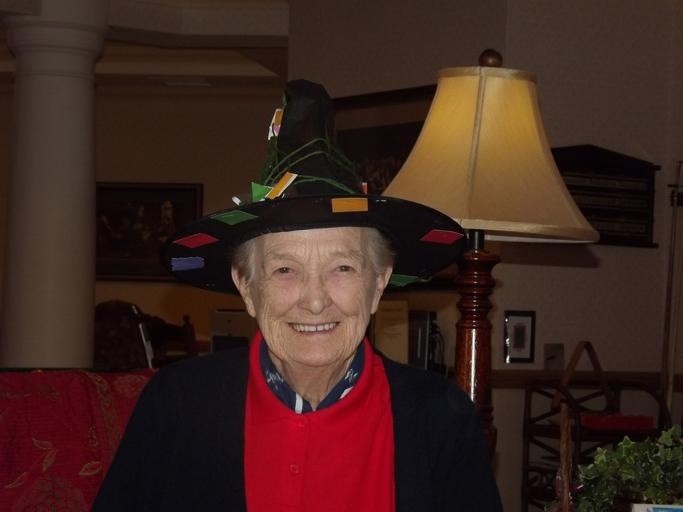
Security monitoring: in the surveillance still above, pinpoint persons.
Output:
[88,226,507,509]
[161,200,173,225]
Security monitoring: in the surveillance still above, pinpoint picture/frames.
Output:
[96,181,203,283]
[503,310,535,364]
[328,82,465,293]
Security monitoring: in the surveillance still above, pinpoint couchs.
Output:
[3,369,148,511]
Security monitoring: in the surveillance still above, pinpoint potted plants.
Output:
[575,423,682,511]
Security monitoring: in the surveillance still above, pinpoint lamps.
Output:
[372,48,603,466]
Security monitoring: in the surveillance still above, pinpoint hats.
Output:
[168,80,467,296]
